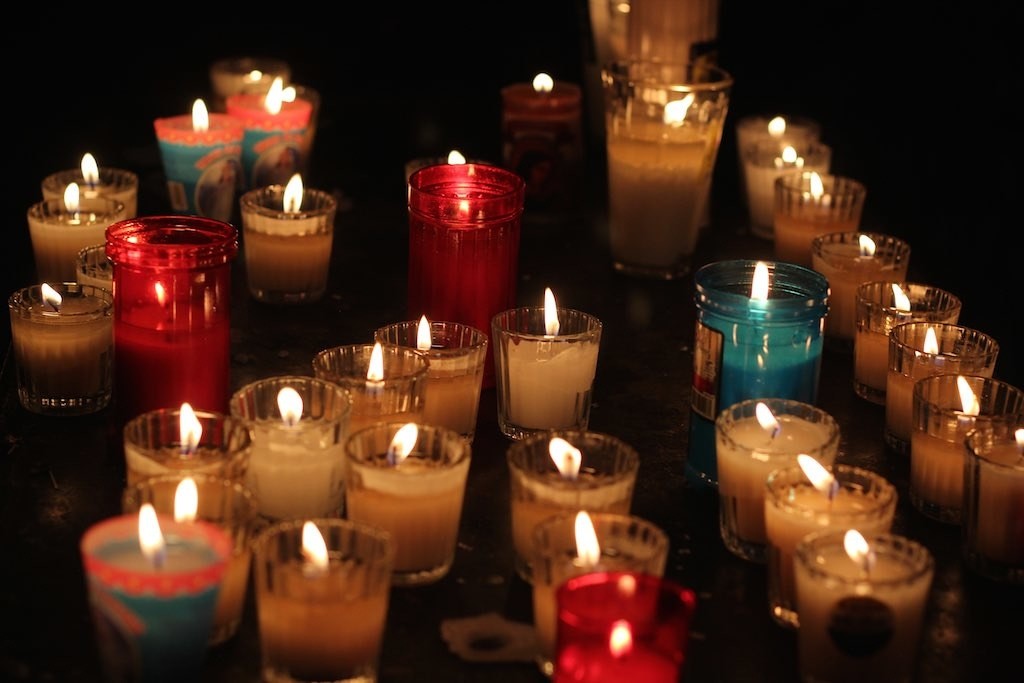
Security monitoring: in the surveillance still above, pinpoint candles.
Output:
[8,0,1024,683]
